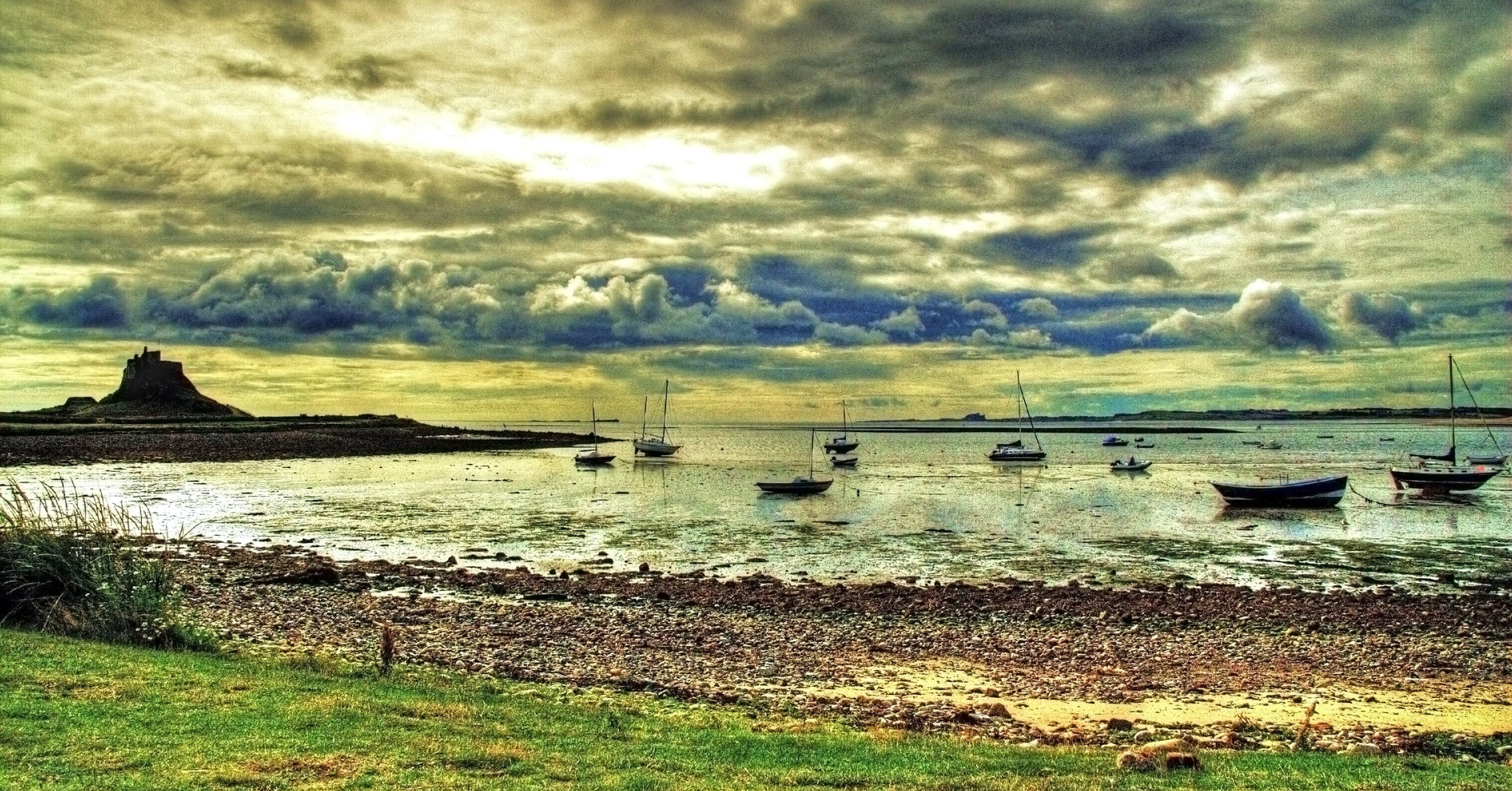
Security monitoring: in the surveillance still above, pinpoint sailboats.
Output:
[823,399,862,455]
[755,426,834,494]
[1389,352,1510,489]
[630,379,685,457]
[988,370,1047,462]
[575,401,617,464]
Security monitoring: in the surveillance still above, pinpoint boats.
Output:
[1109,454,1154,472]
[1242,441,1262,444]
[830,456,860,468]
[1465,455,1509,464]
[1379,438,1395,442]
[1134,443,1156,449]
[1187,437,1205,440]
[1208,474,1350,508]
[1101,436,1130,446]
[1261,440,1282,449]
[1317,434,1334,439]
[1134,437,1144,443]
